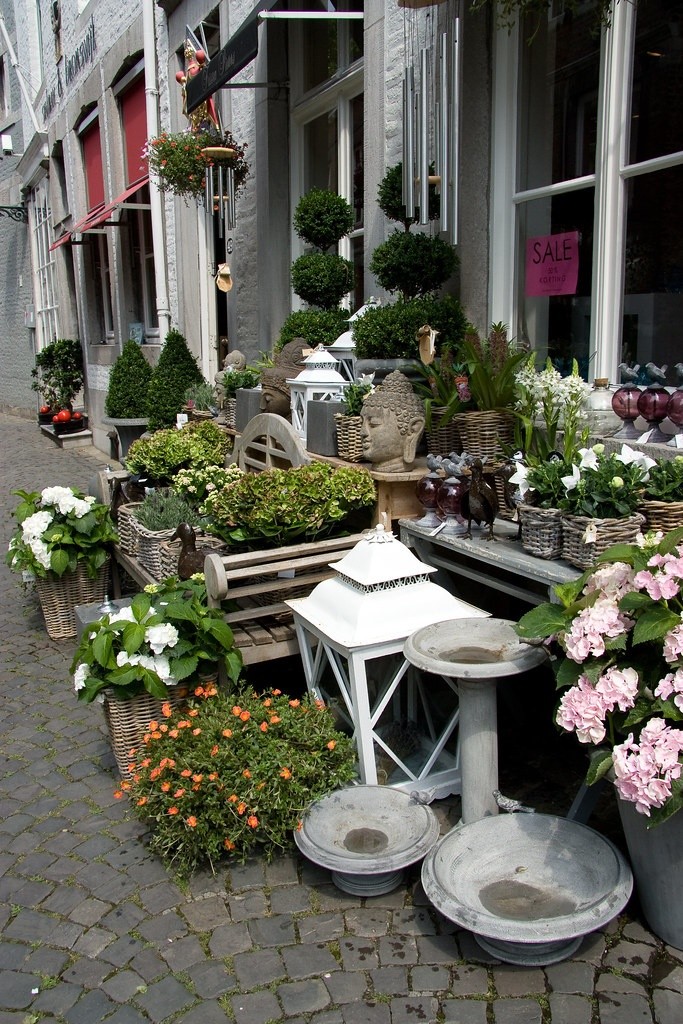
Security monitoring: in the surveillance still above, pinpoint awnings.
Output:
[185,0,364,116]
[47,173,149,250]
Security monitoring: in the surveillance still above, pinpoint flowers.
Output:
[135,124,253,210]
[3,320,683,892]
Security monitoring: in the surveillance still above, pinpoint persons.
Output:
[212,350,247,425]
[359,370,426,473]
[259,338,312,424]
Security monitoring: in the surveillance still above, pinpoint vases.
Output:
[332,411,368,464]
[558,509,645,572]
[99,672,220,786]
[420,405,463,460]
[450,402,522,463]
[634,496,683,548]
[159,535,224,583]
[222,396,236,430]
[606,715,683,952]
[34,553,111,643]
[512,498,565,562]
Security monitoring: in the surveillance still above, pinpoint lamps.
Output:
[284,343,356,450]
[636,362,675,444]
[323,295,385,383]
[665,363,683,447]
[281,519,497,805]
[611,362,644,439]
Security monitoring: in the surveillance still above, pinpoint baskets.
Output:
[560,511,646,571]
[517,501,564,560]
[634,489,683,545]
[192,409,214,422]
[159,537,226,582]
[452,406,516,464]
[118,502,142,556]
[492,462,519,521]
[425,407,463,458]
[181,406,196,421]
[35,552,112,641]
[130,518,203,582]
[224,398,236,429]
[333,412,365,462]
[101,671,218,782]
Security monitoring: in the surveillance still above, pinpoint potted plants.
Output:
[139,327,210,442]
[28,338,74,425]
[50,337,87,436]
[99,336,155,460]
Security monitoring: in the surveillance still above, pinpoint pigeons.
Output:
[107,476,153,525]
[456,458,501,541]
[172,522,250,590]
